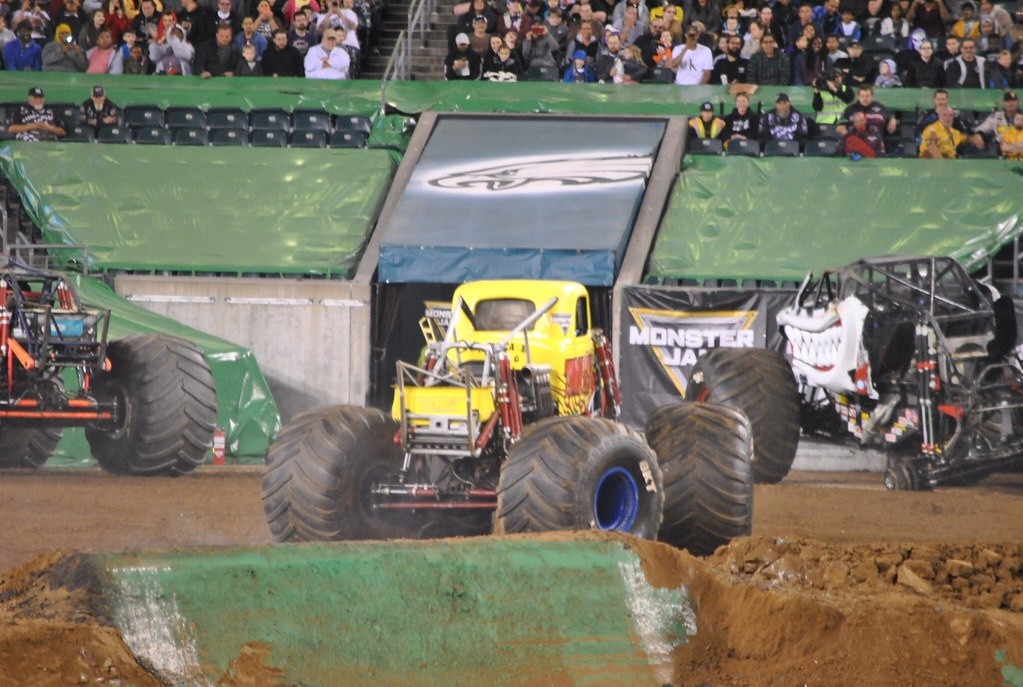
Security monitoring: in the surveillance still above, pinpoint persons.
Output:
[3,17,41,69]
[4,86,66,141]
[304,29,350,79]
[764,93,808,153]
[688,101,728,152]
[192,23,236,76]
[670,23,714,85]
[261,28,306,77]
[42,22,89,72]
[725,91,760,140]
[443,31,482,80]
[810,65,854,124]
[79,86,119,126]
[918,108,983,158]
[1,0,1023,158]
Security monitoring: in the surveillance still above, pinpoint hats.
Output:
[324,29,337,39]
[472,15,488,25]
[1004,91,1018,101]
[774,93,789,104]
[89,87,106,98]
[456,34,470,46]
[701,101,714,111]
[28,88,43,98]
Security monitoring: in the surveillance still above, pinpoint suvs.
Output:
[685,255,1022,490]
[0,260,219,478]
[260,278,755,558]
